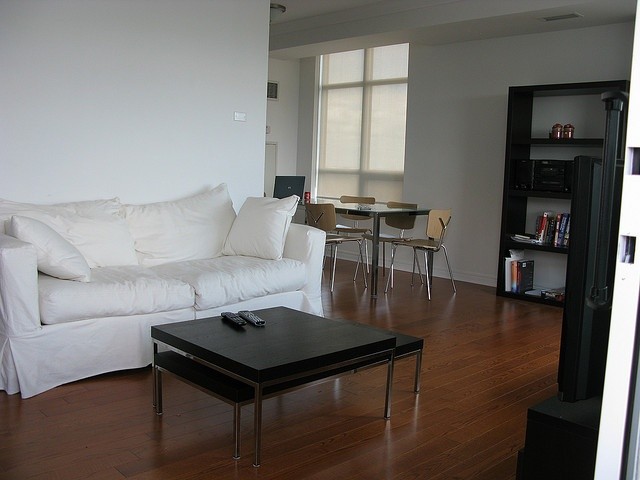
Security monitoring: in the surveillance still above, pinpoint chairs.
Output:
[305,203,370,292]
[355,201,425,294]
[385,209,458,302]
[337,195,374,282]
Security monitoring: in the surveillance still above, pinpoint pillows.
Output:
[0,199,139,268]
[3,215,92,283]
[123,183,236,270]
[223,194,300,261]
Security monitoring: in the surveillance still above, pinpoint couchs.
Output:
[0,181,327,397]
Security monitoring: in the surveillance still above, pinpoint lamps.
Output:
[270,3,286,26]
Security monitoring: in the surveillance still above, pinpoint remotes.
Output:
[221,312,247,326]
[238,311,266,327]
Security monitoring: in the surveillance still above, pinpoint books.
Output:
[506,210,570,248]
[505,257,534,294]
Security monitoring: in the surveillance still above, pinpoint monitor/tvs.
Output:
[556,155,604,401]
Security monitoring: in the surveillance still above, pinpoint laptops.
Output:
[273,175,305,201]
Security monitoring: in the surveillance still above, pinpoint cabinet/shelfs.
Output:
[514,393,601,479]
[493,78,630,311]
